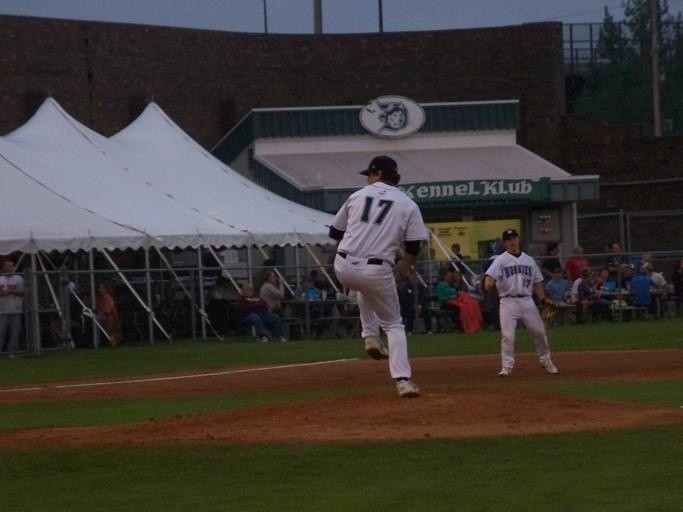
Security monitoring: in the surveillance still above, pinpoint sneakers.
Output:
[540,357,558,374]
[396,378,421,397]
[363,334,389,360]
[497,366,513,377]
[259,333,289,344]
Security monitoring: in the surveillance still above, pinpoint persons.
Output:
[208,270,325,342]
[326,153,428,398]
[477,229,558,377]
[50,272,141,348]
[543,242,682,323]
[1,258,25,358]
[397,243,496,336]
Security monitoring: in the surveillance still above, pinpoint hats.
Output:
[502,228,520,241]
[357,155,398,174]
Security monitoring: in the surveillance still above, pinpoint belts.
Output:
[338,250,383,266]
[505,292,527,298]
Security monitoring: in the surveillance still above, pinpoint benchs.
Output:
[228,289,664,340]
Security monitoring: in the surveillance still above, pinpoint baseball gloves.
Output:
[542,299,559,321]
